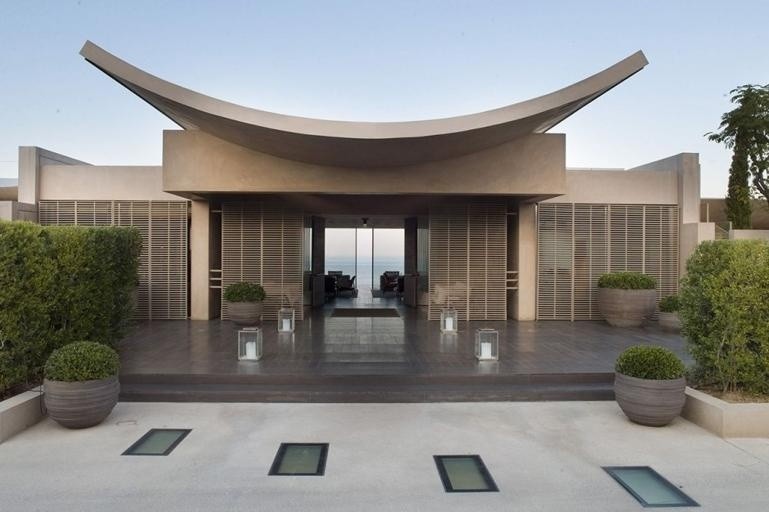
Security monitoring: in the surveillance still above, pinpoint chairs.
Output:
[325,271,356,297]
[380,271,406,301]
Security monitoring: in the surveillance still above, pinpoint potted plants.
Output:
[597,272,656,327]
[659,295,679,328]
[614,344,686,426]
[223,282,266,325]
[43,341,120,430]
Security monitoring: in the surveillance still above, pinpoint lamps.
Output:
[361,218,369,227]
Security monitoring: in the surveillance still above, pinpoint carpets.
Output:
[330,308,400,317]
[371,290,398,298]
[325,289,358,298]
